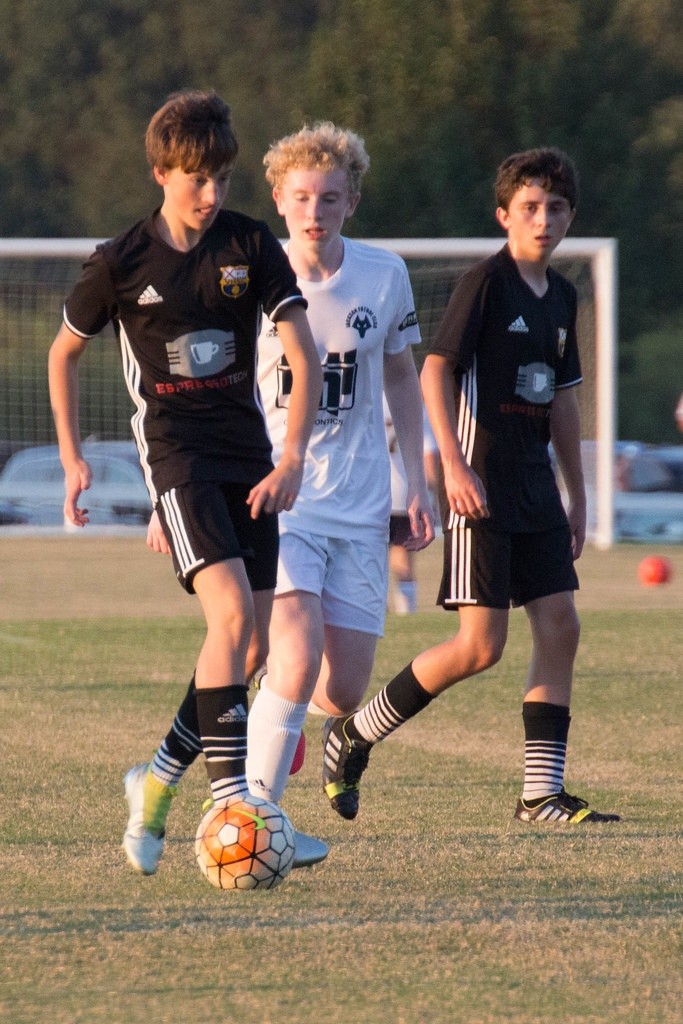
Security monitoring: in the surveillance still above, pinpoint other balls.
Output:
[636,553,675,587]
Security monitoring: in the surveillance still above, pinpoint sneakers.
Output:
[514,786,620,824]
[125,763,178,878]
[291,832,327,869]
[322,710,374,821]
[253,669,267,693]
[393,580,417,615]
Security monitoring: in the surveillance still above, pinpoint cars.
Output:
[0,436,153,527]
[646,445,683,481]
[556,442,683,544]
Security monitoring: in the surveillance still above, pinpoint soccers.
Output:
[191,791,298,895]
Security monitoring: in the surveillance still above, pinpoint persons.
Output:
[45,94,334,875]
[322,150,628,830]
[246,121,436,870]
